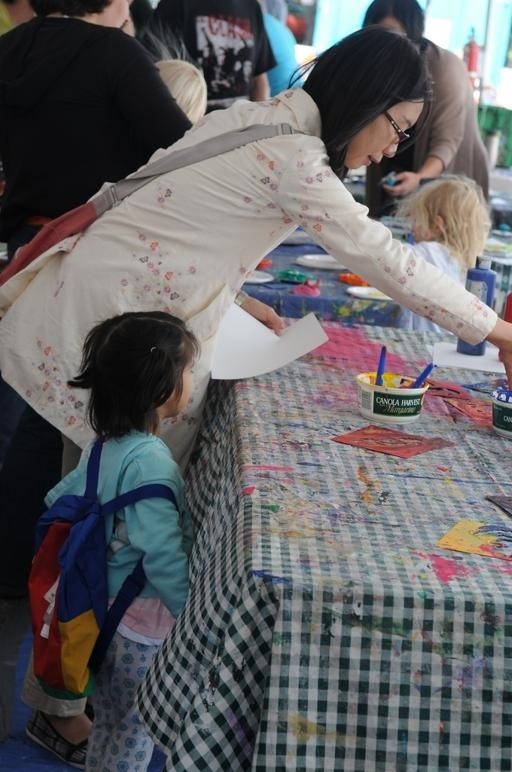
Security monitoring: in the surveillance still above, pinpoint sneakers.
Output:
[25,709,87,770]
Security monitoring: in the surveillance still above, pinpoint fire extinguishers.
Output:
[465,28,479,72]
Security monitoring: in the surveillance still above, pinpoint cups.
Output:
[355,372,429,423]
[491,397,511,440]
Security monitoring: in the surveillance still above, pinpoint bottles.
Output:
[456,257,496,356]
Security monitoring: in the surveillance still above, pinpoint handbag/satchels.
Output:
[1,201,97,287]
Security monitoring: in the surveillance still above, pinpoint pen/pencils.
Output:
[413,363,434,387]
[376,347,386,385]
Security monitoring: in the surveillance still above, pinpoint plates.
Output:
[244,270,274,284]
[345,283,391,302]
[295,251,347,271]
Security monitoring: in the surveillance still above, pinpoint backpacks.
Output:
[27,440,176,698]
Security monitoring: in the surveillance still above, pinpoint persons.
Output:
[44,310,201,772]
[394,173,491,332]
[0,0,302,265]
[357,0,489,221]
[0,29,512,767]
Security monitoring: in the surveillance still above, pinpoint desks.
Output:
[228,246,413,328]
[224,318,511,772]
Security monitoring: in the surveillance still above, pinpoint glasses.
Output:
[384,114,410,143]
